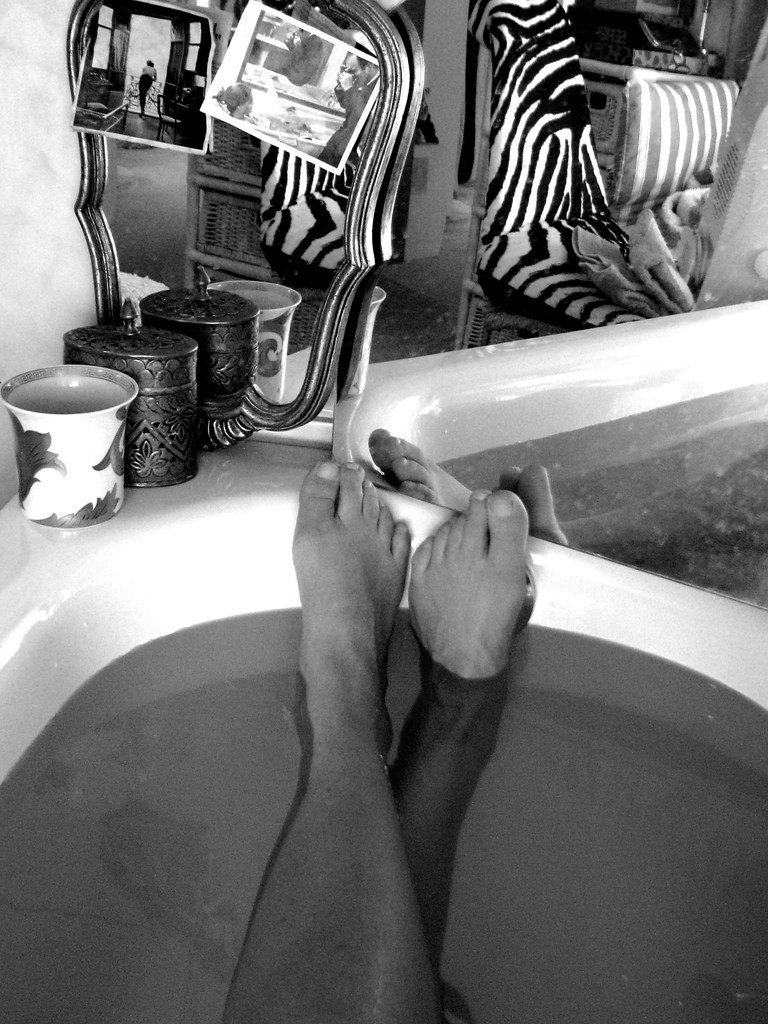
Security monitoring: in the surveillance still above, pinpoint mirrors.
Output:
[69,0,409,450]
[330,0,768,612]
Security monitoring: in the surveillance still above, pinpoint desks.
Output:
[77,94,130,135]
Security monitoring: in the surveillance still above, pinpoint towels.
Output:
[571,207,693,319]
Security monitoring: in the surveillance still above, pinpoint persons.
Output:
[368,428,768,592]
[283,26,324,87]
[222,457,529,1024]
[214,84,256,124]
[316,54,381,168]
[138,59,157,117]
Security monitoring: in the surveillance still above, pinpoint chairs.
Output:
[155,92,184,145]
[184,118,327,350]
[453,1,663,352]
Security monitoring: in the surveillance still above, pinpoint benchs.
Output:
[612,75,739,222]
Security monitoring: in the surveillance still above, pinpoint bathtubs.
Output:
[348,293,768,609]
[0,439,768,1024]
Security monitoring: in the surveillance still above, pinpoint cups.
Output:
[346,284,387,397]
[207,280,303,404]
[0,365,138,529]
